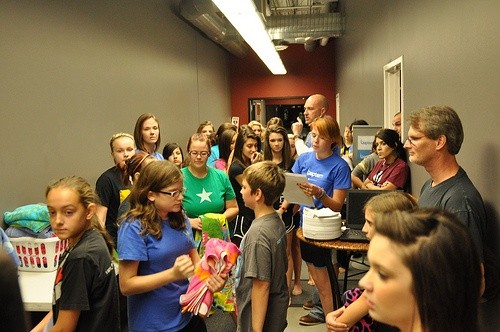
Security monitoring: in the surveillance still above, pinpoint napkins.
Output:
[308,207,339,218]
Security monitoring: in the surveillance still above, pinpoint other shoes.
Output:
[303,300,316,310]
[298,315,322,325]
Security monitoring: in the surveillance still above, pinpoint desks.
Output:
[296,227,370,310]
[18,262,121,332]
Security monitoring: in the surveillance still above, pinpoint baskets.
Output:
[3,235,69,271]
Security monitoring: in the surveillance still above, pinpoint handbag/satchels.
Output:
[344,287,374,332]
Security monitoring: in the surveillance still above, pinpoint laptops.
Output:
[341,189,405,240]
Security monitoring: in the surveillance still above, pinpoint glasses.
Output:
[407,135,432,146]
[158,187,186,197]
[189,150,210,157]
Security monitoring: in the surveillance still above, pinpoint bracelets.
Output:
[293,135,300,141]
[316,188,326,200]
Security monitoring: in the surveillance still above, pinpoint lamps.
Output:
[273,39,289,51]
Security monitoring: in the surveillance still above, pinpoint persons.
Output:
[117,152,158,216]
[162,143,184,169]
[133,113,164,162]
[392,112,401,138]
[115,161,229,332]
[362,128,408,190]
[229,129,262,247]
[341,120,368,158]
[292,94,329,156]
[403,105,485,259]
[261,124,303,295]
[199,116,284,169]
[351,129,386,189]
[180,132,239,239]
[325,191,419,332]
[288,133,316,286]
[235,161,290,332]
[358,207,483,332]
[93,133,136,248]
[291,115,352,325]
[29,176,120,332]
